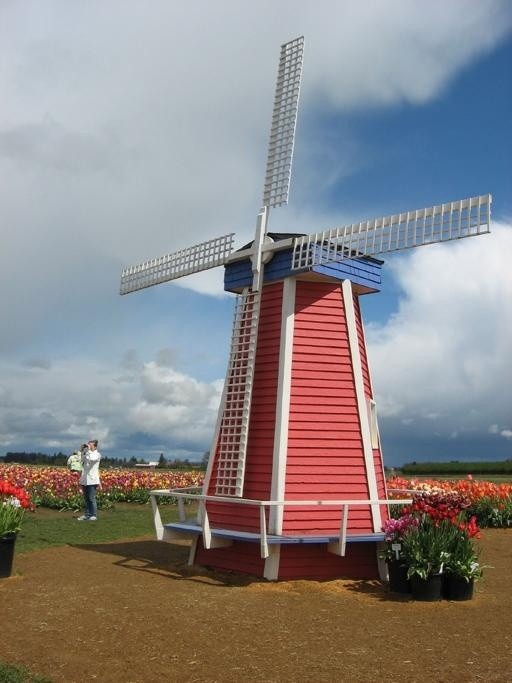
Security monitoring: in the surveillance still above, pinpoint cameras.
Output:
[82,444,88,448]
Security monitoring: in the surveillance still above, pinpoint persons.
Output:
[67,449,83,475]
[77,439,102,520]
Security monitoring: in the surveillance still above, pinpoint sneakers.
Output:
[77,514,98,521]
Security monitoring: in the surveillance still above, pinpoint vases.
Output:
[386,553,473,600]
[0,532,16,576]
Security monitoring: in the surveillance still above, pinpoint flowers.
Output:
[0,481,30,537]
[377,488,494,584]
